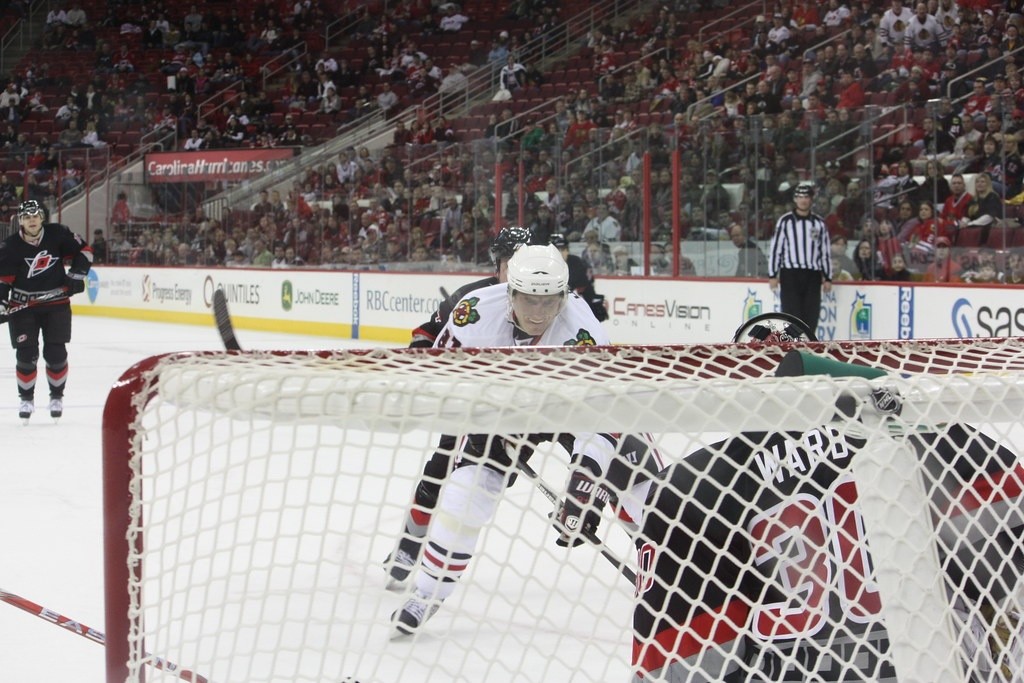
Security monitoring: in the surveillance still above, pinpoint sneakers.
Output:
[18,400,35,427]
[50,399,63,424]
[382,549,416,590]
[389,588,445,641]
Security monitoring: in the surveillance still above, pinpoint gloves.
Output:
[556,472,612,547]
[468,432,511,460]
[408,334,434,348]
[62,271,84,297]
[0,300,10,324]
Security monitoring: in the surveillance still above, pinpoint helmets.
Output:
[548,234,569,246]
[730,313,819,343]
[506,242,569,296]
[18,200,43,219]
[491,228,532,256]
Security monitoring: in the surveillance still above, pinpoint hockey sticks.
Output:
[206,288,243,351]
[1,592,208,683]
[504,442,638,589]
[0,285,71,317]
[438,287,458,311]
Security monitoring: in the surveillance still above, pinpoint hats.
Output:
[982,9,994,16]
[756,16,766,22]
[803,58,814,63]
[773,13,783,18]
[795,185,814,197]
[995,74,1003,79]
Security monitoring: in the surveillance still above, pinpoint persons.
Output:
[0,0,1024,279]
[0,201,92,417]
[770,187,831,339]
[384,225,664,633]
[632,313,1024,682]
